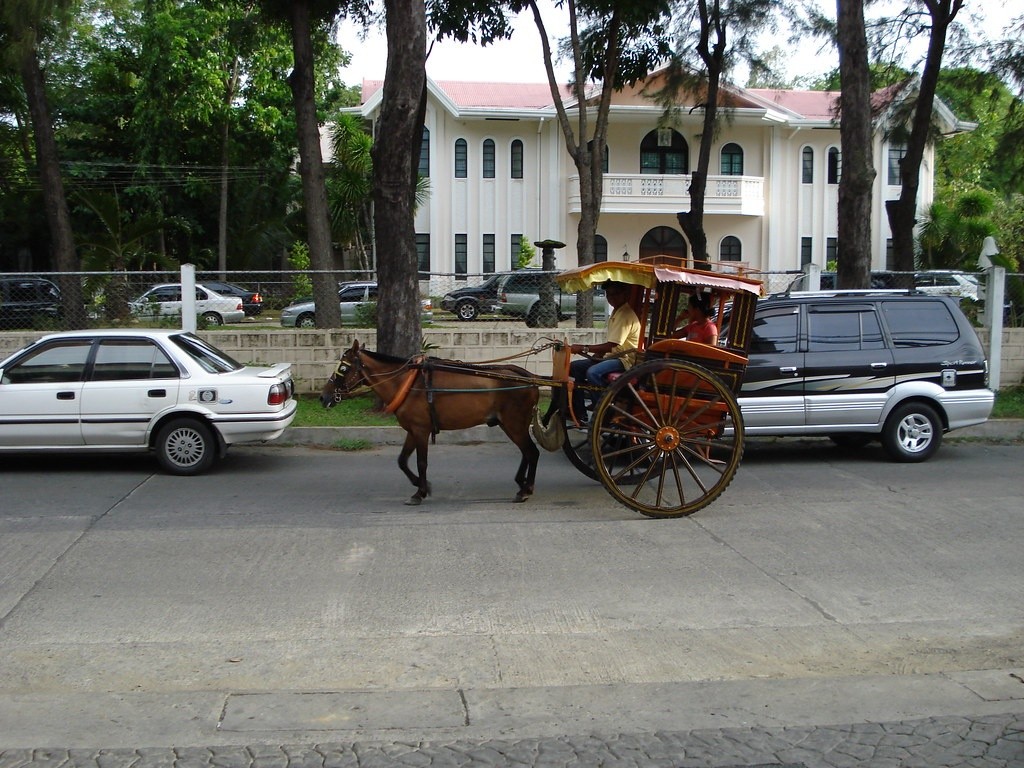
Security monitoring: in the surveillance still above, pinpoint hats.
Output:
[601,278,631,289]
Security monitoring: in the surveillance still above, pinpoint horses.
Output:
[319,339,541,506]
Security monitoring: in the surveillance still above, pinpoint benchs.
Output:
[606,372,639,387]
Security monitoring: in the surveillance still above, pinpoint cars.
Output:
[278,280,433,330]
[124,284,246,329]
[199,280,263,317]
[0,328,297,476]
[914,268,986,302]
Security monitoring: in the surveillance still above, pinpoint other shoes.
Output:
[586,403,594,412]
[575,412,589,421]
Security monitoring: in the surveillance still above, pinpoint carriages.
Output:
[318,255,766,521]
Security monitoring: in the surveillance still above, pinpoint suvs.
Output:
[488,268,610,328]
[0,274,69,329]
[575,288,995,469]
[438,271,514,321]
[786,267,910,294]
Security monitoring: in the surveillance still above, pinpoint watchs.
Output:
[582,345,589,354]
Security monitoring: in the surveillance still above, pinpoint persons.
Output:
[669,292,718,348]
[569,278,644,421]
